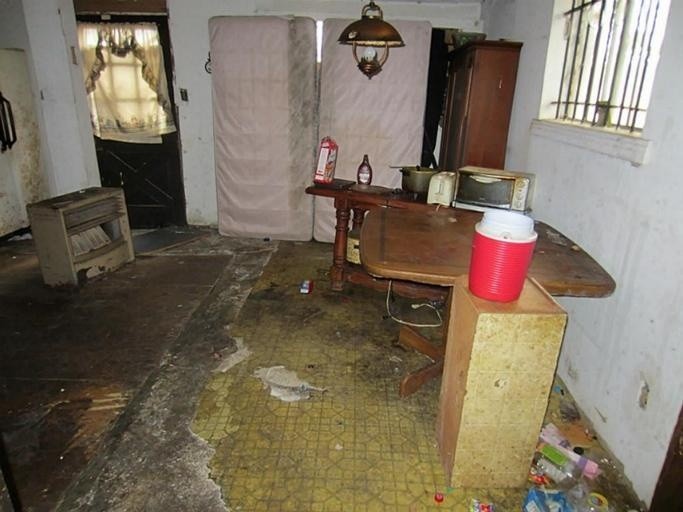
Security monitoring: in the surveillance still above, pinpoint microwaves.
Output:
[450,164,536,218]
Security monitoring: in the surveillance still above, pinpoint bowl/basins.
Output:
[450,31,486,49]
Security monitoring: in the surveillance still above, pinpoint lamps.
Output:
[337,0,405,81]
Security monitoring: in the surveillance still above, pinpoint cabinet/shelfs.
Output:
[27,187,135,290]
[438,40,522,171]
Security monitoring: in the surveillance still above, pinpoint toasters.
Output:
[425,170,456,208]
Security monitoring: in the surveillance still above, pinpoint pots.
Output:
[400,164,439,195]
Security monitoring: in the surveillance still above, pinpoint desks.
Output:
[306,178,449,306]
[361,208,616,399]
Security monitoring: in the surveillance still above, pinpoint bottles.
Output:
[355,154,372,185]
[531,437,582,490]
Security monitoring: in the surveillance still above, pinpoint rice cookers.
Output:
[344,230,362,266]
